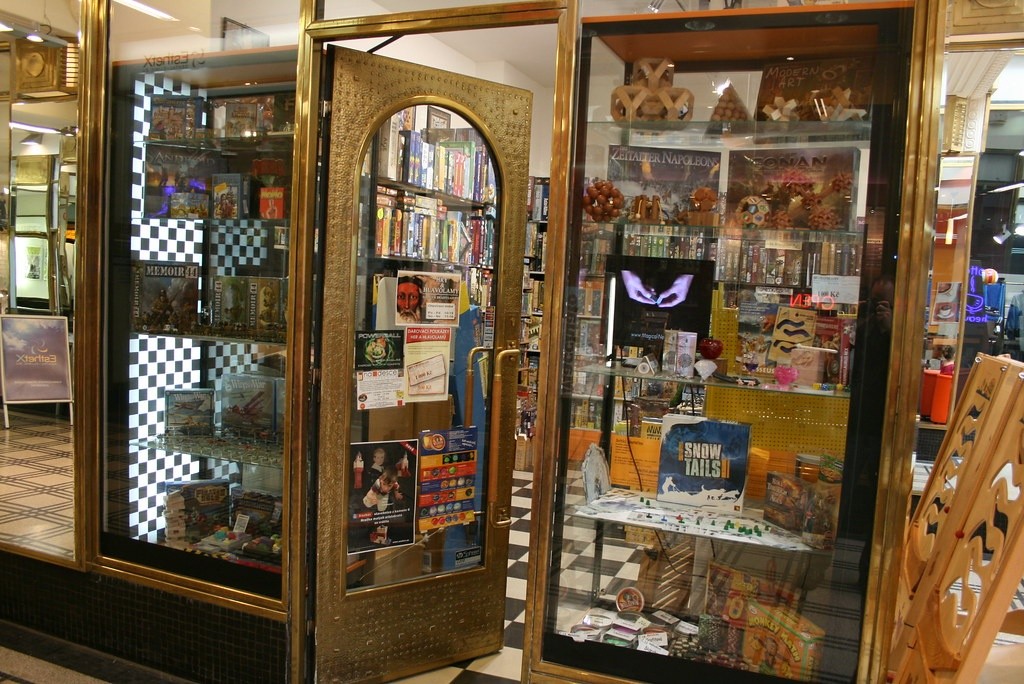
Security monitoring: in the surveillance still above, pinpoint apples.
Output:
[700,335,723,359]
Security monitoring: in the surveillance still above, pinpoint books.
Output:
[516,174,862,550]
[354,106,497,411]
[349,426,483,569]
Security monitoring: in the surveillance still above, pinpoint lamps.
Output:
[19,132,45,146]
[13,35,79,100]
[993,223,1011,245]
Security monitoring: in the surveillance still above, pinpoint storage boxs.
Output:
[149,87,297,139]
[706,564,826,682]
[169,173,286,221]
[764,471,814,529]
[131,259,282,569]
[363,520,480,583]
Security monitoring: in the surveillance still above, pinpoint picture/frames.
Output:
[222,16,272,51]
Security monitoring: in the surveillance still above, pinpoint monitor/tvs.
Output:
[598,253,716,367]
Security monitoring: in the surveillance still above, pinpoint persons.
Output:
[1004,295,1024,357]
[930,345,956,377]
[618,259,699,308]
[368,449,386,483]
[363,469,403,513]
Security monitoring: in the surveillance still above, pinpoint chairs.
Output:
[580,443,662,605]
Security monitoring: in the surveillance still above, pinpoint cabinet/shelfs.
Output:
[10,99,78,343]
[914,150,1024,429]
[360,113,869,502]
[99,42,298,605]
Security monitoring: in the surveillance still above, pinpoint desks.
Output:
[575,488,813,627]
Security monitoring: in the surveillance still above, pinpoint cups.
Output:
[795,453,822,483]
[931,373,952,423]
[661,327,698,378]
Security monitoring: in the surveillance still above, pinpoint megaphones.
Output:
[659,328,697,376]
